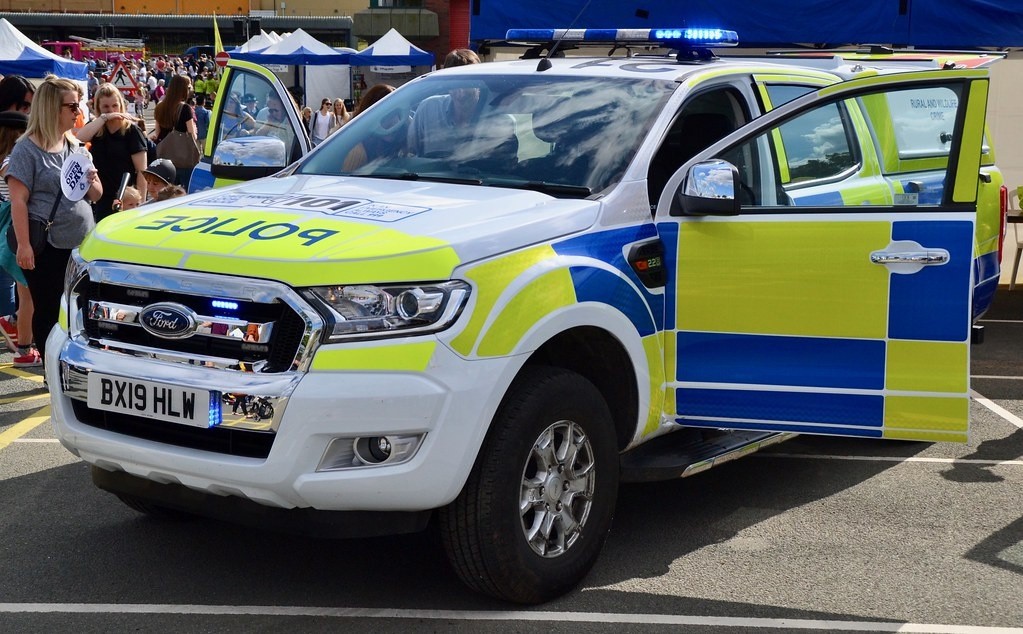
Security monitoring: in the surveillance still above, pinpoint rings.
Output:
[19,262,21,263]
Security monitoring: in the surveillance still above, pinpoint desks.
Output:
[1007,209,1023,223]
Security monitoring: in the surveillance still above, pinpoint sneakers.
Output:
[0,317,19,352]
[14,348,43,367]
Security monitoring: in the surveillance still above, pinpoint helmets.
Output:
[141,158,176,186]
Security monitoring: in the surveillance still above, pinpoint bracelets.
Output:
[101,113,108,121]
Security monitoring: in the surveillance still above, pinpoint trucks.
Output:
[40,35,146,63]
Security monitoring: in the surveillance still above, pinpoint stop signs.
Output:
[215,51,230,67]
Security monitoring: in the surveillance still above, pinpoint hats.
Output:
[243,93,257,103]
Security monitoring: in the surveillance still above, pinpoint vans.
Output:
[178,45,236,64]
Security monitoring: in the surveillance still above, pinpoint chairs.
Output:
[645,111,703,205]
[507,105,578,182]
[1008,191,1023,290]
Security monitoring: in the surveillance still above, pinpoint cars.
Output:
[44,45,1009,604]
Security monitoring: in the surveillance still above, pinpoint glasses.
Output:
[61,103,79,113]
[325,103,332,106]
[268,109,279,114]
[19,101,31,110]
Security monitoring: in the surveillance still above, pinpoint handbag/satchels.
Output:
[156,103,200,169]
[7,212,48,257]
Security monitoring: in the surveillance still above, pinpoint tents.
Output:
[225,26,436,110]
[0,18,90,124]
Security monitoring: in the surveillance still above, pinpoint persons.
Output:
[218,83,413,173]
[409,47,518,158]
[0,50,225,368]
